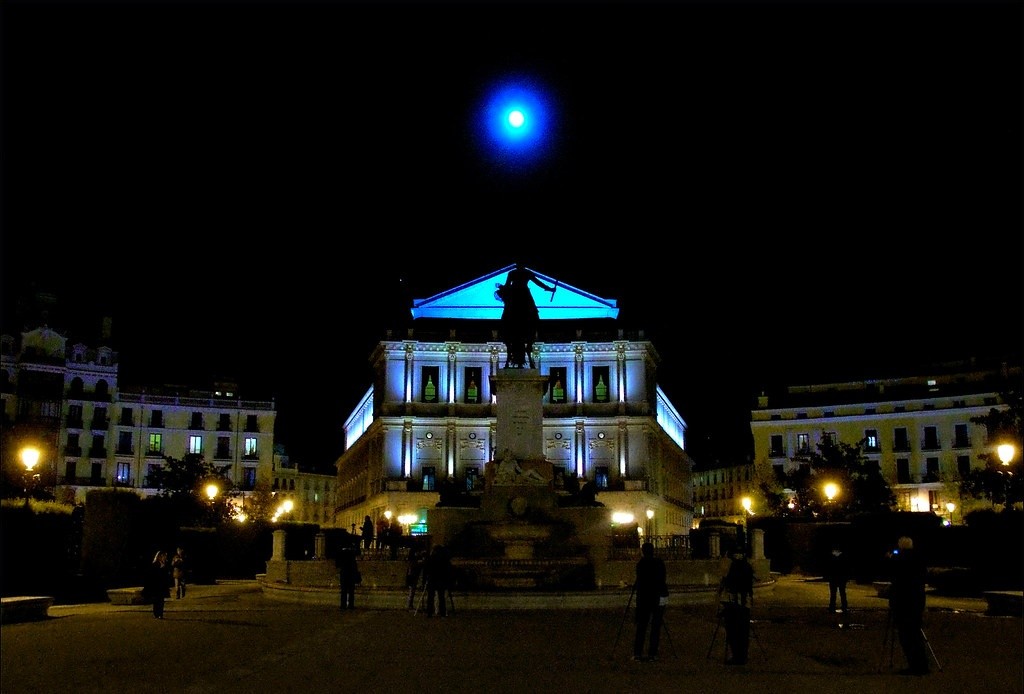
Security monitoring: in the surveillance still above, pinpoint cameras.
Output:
[892,550,899,555]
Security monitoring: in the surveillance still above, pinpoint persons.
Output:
[499,449,552,483]
[721,547,754,666]
[823,548,850,619]
[630,542,667,663]
[151,548,187,620]
[335,516,451,618]
[505,263,556,351]
[885,536,931,676]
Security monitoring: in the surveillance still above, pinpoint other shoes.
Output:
[630,656,642,665]
[647,656,655,664]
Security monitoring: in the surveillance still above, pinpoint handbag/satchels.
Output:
[406,575,417,585]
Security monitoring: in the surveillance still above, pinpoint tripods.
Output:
[707,619,767,666]
[609,587,678,660]
[413,580,455,616]
[878,607,942,675]
